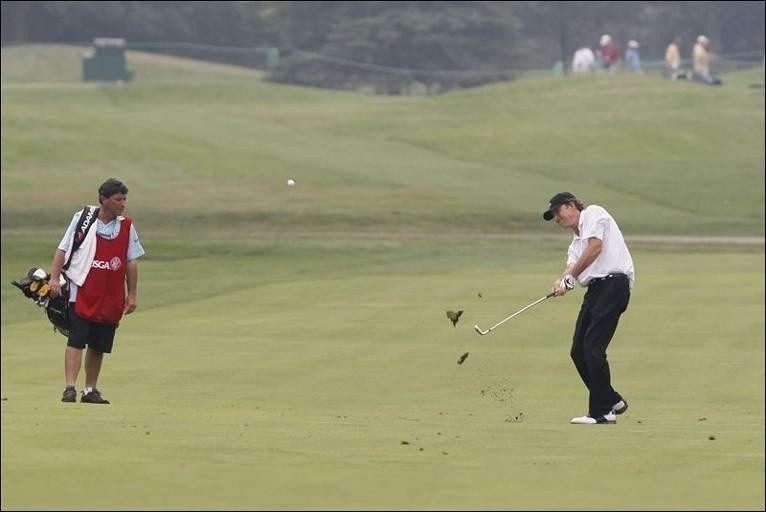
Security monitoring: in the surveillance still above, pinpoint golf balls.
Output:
[286,179,295,187]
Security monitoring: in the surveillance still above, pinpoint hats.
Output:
[543,192,578,221]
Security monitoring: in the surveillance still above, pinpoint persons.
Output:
[45,178,144,404]
[571,32,716,89]
[543,192,636,424]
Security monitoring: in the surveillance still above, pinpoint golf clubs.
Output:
[475,291,556,335]
[12,264,67,309]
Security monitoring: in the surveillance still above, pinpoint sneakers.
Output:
[571,400,628,425]
[61,386,76,402]
[81,387,109,404]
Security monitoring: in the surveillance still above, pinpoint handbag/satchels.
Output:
[46,292,70,332]
